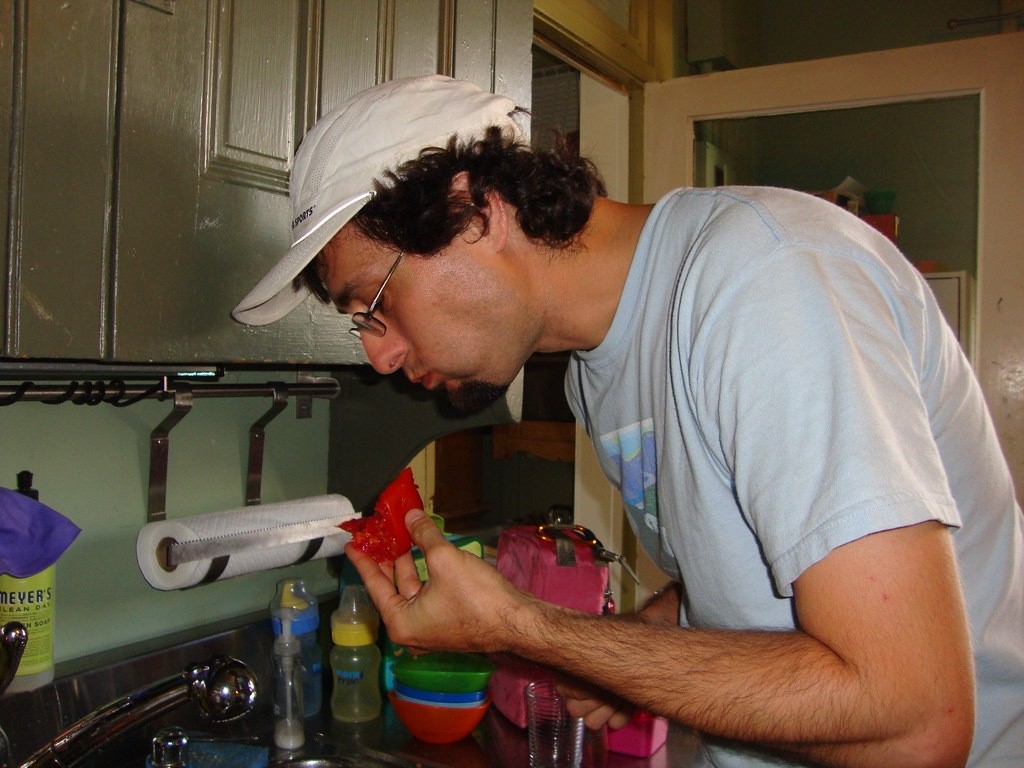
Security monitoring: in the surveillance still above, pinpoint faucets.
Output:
[16,657,259,767]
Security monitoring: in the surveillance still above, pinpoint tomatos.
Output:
[337,467,427,563]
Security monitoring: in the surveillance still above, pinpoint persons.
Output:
[234,75,1024,768]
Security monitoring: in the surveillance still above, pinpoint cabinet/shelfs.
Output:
[0,0,533,386]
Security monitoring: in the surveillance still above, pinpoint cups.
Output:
[525,680,607,768]
[864,188,898,214]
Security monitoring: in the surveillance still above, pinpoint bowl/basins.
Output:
[388,659,494,744]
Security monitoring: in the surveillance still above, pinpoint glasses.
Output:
[348,249,408,341]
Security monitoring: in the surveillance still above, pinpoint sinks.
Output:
[266,736,434,768]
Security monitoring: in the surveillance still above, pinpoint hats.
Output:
[230,75,524,327]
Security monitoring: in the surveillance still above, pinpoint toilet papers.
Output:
[137,493,364,591]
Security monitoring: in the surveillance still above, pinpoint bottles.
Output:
[268,578,322,719]
[0,470,57,695]
[269,607,306,751]
[328,585,383,723]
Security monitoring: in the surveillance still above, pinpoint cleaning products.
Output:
[0,470,58,694]
